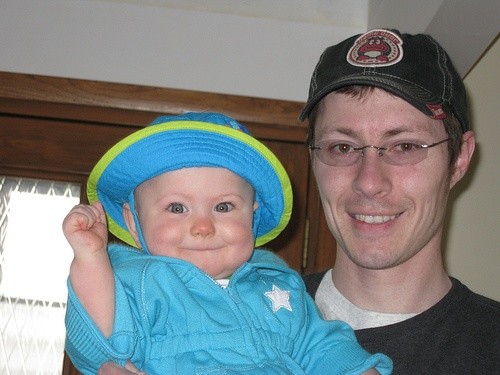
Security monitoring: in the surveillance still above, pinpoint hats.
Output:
[298,29,469,133]
[86,112,294,251]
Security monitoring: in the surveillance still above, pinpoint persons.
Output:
[62,111,396,375]
[94,28,500,374]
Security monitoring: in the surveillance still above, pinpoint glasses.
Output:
[308,138,450,167]
